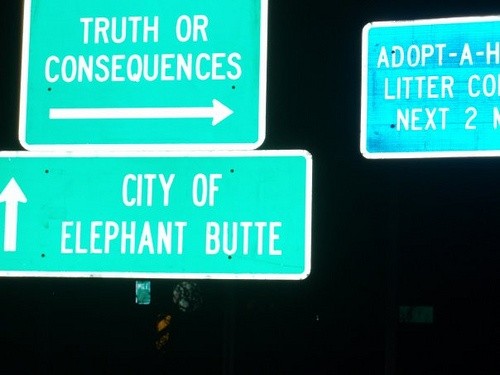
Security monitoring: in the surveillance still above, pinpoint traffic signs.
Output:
[18,1,268,149]
[359,16,500,161]
[0,149,312,280]
